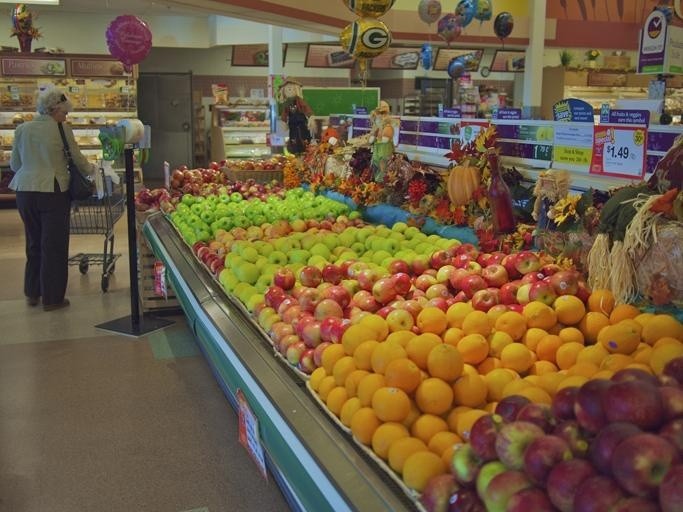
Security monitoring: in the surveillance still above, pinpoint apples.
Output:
[423,356,683,512]
[134,155,592,374]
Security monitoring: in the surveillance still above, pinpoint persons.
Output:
[8,86,93,311]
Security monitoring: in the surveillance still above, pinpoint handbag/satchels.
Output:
[69,164,96,201]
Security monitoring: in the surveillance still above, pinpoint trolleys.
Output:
[66,168,124,293]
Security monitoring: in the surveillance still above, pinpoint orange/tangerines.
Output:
[310,287,683,492]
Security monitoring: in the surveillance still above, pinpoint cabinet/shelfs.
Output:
[209,99,274,172]
[0,75,150,209]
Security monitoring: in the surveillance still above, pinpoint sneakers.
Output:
[26,295,41,305]
[42,299,70,311]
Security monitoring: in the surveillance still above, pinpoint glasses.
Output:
[57,94,67,105]
